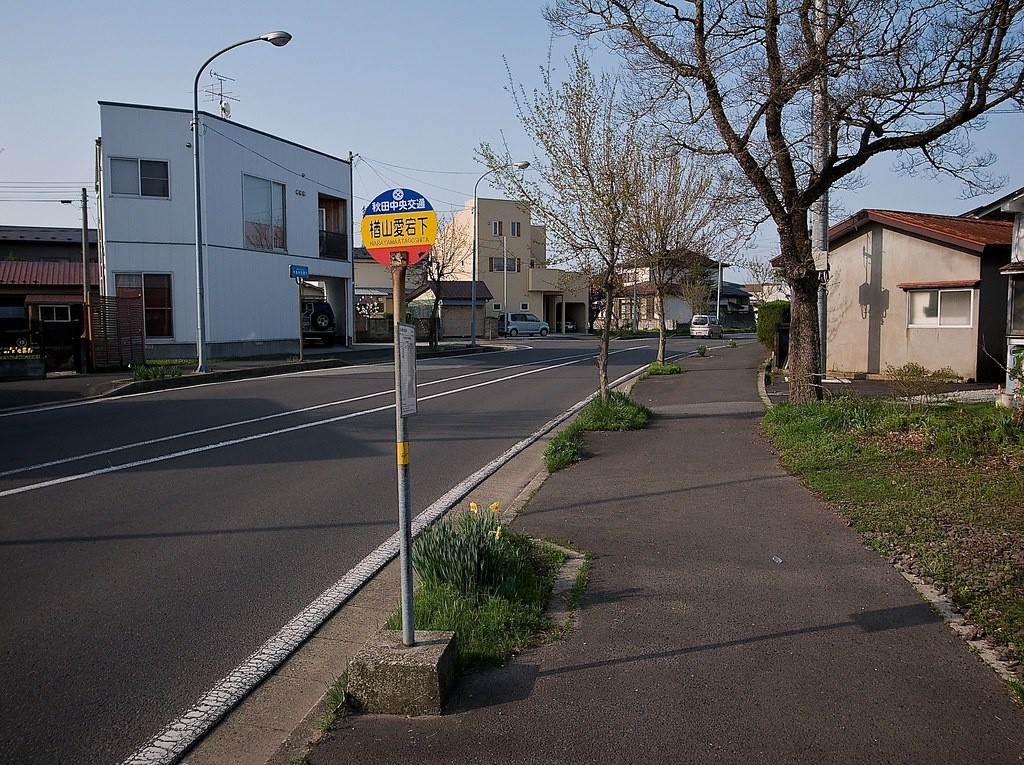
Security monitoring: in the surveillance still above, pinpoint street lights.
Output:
[193,30,292,374]
[717,237,751,321]
[472,161,531,347]
[61,200,94,373]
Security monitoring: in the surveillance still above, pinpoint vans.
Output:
[497,312,549,336]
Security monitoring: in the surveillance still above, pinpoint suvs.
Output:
[301,296,337,346]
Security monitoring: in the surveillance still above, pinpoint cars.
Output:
[689,315,724,339]
[557,319,578,333]
